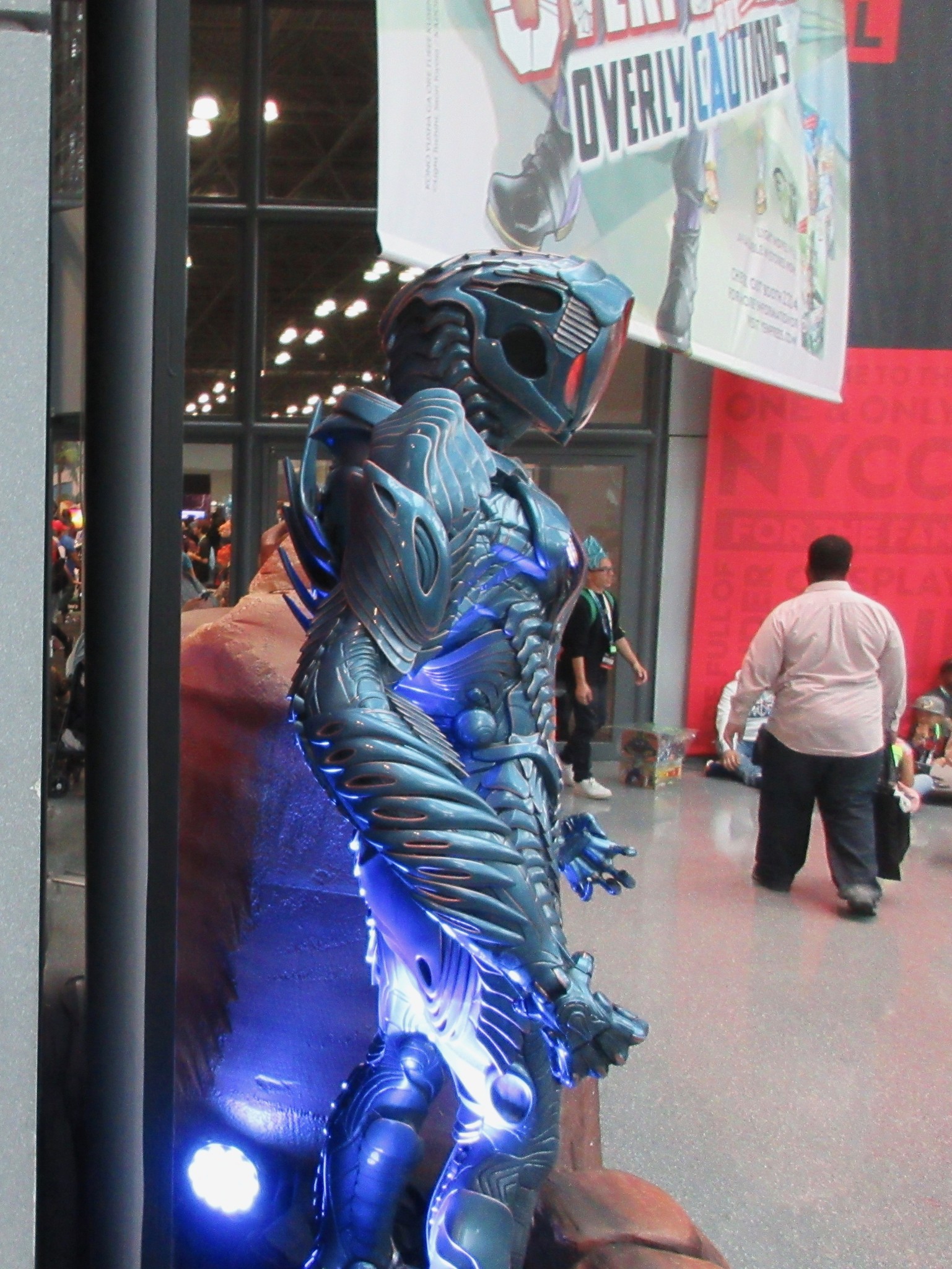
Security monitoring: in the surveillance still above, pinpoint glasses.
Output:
[590,564,613,573]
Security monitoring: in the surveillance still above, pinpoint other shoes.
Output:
[705,759,724,776]
[753,864,794,891]
[851,891,879,917]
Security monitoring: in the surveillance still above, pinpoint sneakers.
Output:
[558,757,612,799]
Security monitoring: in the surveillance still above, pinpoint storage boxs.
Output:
[617,727,683,791]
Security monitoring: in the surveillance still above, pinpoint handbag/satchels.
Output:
[875,787,912,881]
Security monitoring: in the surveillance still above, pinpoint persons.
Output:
[873,658,952,816]
[556,552,648,799]
[723,535,906,917]
[44,497,86,797]
[278,249,649,1269]
[704,668,776,788]
[276,500,292,523]
[181,505,233,609]
